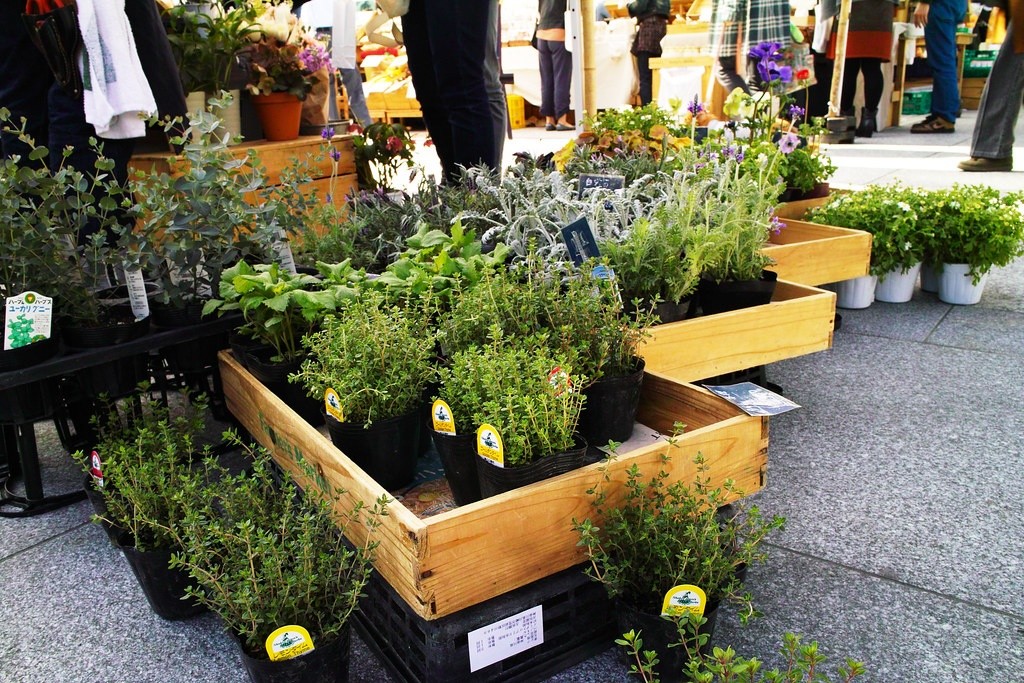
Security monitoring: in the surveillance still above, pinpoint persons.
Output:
[825,0,896,138]
[382,0,506,190]
[957,0,1024,174]
[625,0,670,109]
[706,0,792,96]
[534,0,576,133]
[300,0,373,131]
[910,0,968,134]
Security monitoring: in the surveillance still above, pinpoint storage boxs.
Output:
[963,49,1000,78]
[216,346,768,620]
[506,95,525,130]
[901,92,932,115]
[688,365,767,391]
[775,188,850,220]
[255,442,629,683]
[961,78,986,109]
[632,279,836,384]
[759,216,873,287]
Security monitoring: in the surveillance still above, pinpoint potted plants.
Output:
[0,0,865,683]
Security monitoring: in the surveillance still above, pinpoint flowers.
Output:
[245,16,1024,287]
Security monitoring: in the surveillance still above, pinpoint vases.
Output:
[251,91,302,142]
[825,261,988,305]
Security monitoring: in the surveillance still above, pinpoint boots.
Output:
[855,106,878,138]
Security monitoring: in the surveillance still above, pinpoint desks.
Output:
[853,22,925,128]
[914,32,978,100]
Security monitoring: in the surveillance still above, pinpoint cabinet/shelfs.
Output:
[127,134,358,256]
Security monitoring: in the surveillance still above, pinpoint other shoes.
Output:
[546,123,576,131]
[910,113,955,133]
[957,157,1013,172]
